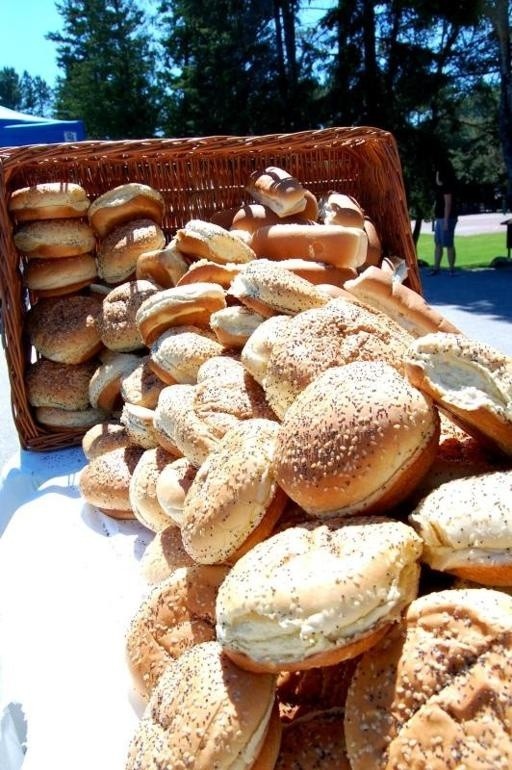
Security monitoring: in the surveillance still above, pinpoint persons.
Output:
[425,156,458,277]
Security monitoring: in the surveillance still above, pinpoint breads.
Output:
[7,165,512,770]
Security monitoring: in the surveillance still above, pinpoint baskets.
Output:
[0,125,423,452]
